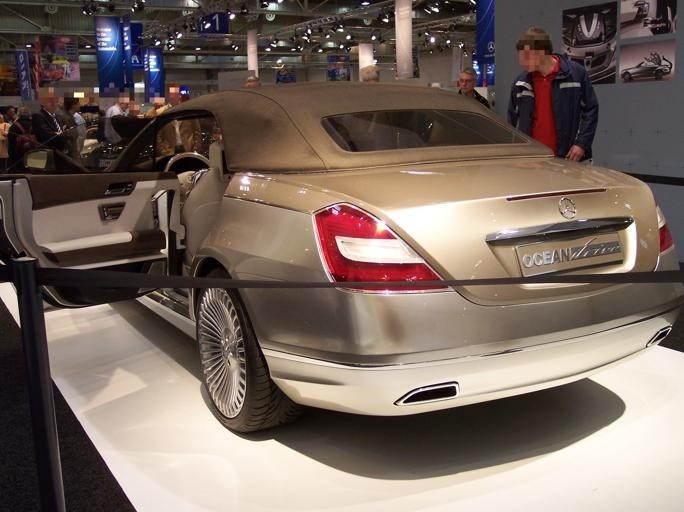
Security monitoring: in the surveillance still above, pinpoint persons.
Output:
[327,55,350,81]
[457,67,490,109]
[359,65,380,84]
[646,53,655,64]
[0,75,264,175]
[62,60,71,78]
[505,26,599,167]
[653,53,662,65]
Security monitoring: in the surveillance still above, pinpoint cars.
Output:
[560,1,673,81]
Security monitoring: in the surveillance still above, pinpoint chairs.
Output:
[180,140,234,267]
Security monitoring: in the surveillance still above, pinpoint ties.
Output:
[53,117,61,131]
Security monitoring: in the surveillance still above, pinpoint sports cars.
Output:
[1,79,681,442]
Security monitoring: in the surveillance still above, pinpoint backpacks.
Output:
[15,122,37,153]
